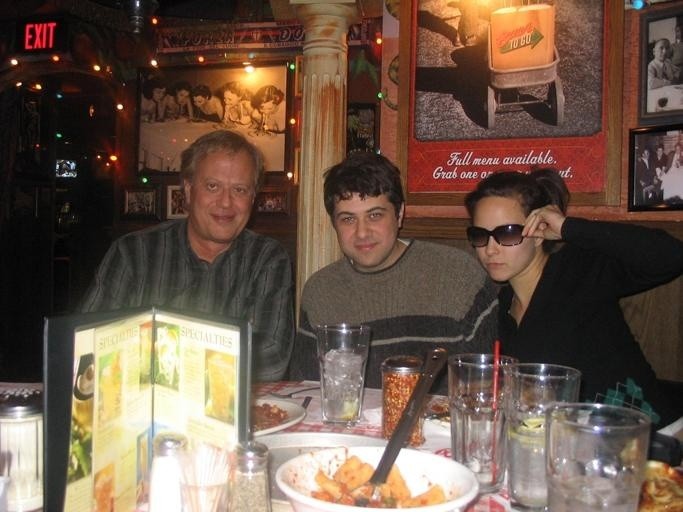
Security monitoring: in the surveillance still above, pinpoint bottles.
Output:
[233,439,271,511]
[149,433,186,511]
[381,354,425,448]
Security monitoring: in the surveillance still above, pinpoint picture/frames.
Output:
[639,4,683,127]
[347,100,380,159]
[122,187,163,221]
[629,124,683,211]
[396,1,625,208]
[134,56,294,178]
[253,185,297,219]
[163,182,189,219]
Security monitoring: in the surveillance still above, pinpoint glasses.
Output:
[465,223,526,248]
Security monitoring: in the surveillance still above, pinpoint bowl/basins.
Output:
[275,445,480,512]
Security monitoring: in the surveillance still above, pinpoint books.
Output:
[42,305,252,512]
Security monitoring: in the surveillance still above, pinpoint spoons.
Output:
[352,345,449,502]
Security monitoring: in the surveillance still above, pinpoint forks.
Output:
[267,386,322,399]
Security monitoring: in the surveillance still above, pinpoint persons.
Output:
[257,193,288,212]
[465,167,683,430]
[191,84,224,122]
[668,24,683,66]
[636,148,656,205]
[251,85,285,133]
[126,192,153,214]
[167,81,194,119]
[648,38,683,90]
[652,145,669,177]
[76,130,295,383]
[172,192,187,215]
[140,77,167,122]
[289,149,497,396]
[667,143,683,173]
[222,80,254,127]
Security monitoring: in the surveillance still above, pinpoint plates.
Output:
[252,430,390,506]
[250,397,307,437]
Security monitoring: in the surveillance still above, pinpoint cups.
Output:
[317,322,372,424]
[444,352,520,495]
[543,403,652,512]
[502,363,582,511]
